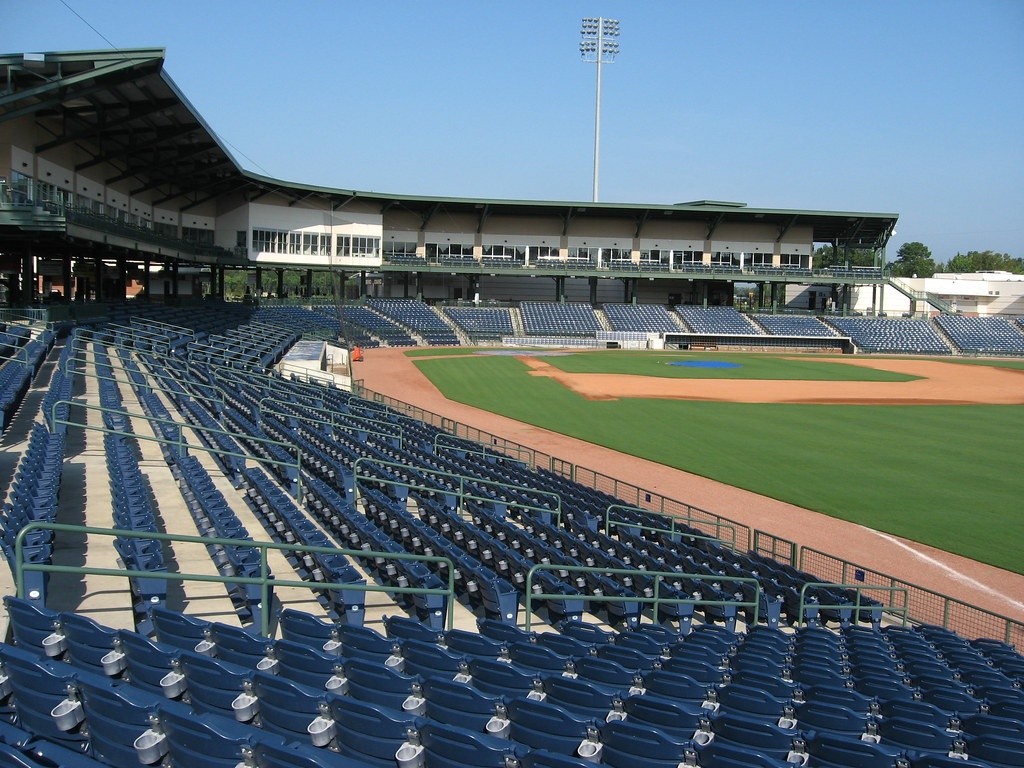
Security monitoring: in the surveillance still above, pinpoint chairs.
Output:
[0,184,1024,767]
